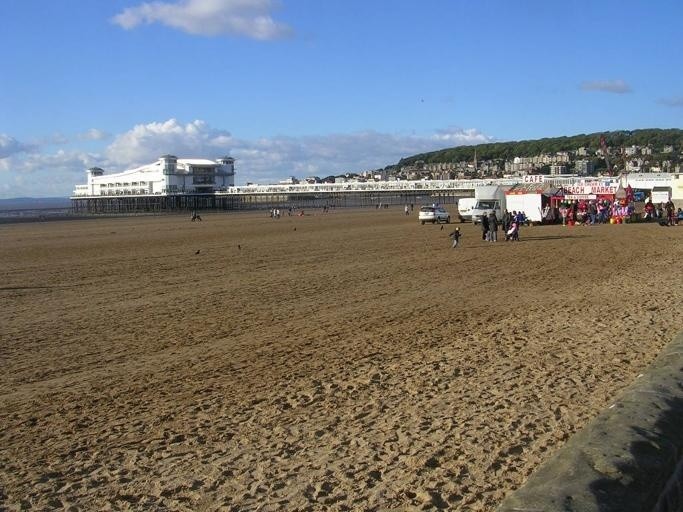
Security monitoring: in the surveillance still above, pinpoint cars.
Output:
[418,205,451,225]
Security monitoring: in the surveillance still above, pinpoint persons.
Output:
[480,208,527,240]
[374,201,389,209]
[410,200,415,211]
[271,206,306,218]
[542,199,683,226]
[449,227,464,248]
[404,203,409,217]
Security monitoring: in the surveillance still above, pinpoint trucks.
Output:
[471,185,506,225]
